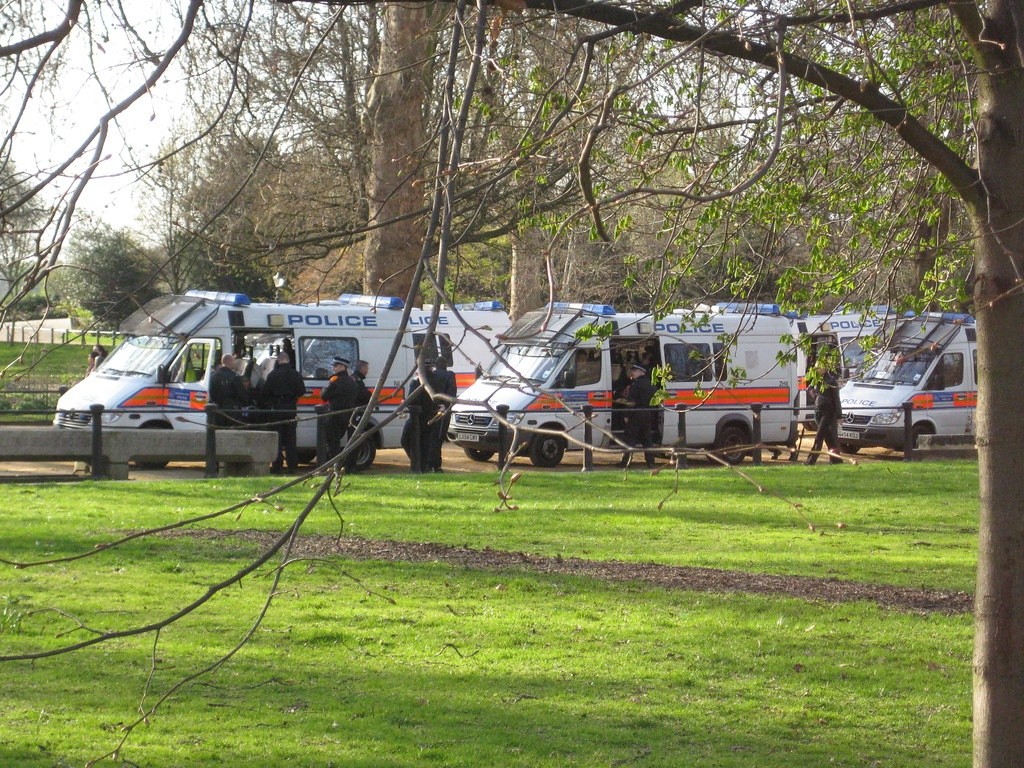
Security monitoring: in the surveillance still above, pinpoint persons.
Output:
[320,356,379,476]
[209,351,306,475]
[85,345,109,378]
[615,345,661,469]
[401,357,458,475]
[804,370,844,465]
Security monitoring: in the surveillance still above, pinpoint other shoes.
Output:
[616,461,629,468]
[801,461,815,465]
[421,465,433,473]
[647,462,655,468]
[434,466,443,473]
[270,466,283,474]
[286,467,296,474]
[829,460,843,464]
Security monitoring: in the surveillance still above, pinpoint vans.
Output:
[792,304,909,431]
[52,287,415,478]
[240,294,516,448]
[444,302,800,468]
[833,309,978,460]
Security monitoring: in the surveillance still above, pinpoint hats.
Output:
[424,359,432,366]
[330,356,351,367]
[630,362,648,373]
